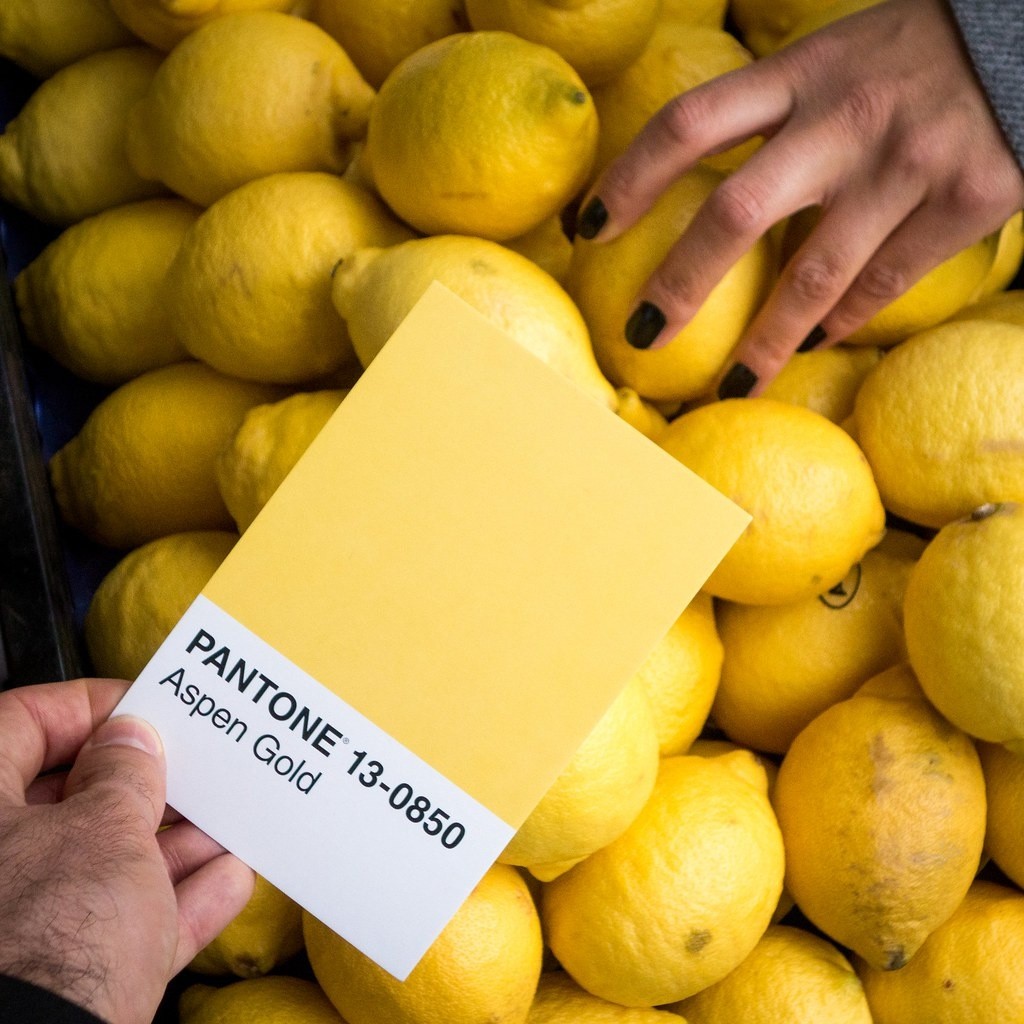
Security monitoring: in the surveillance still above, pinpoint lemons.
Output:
[0,0,1024,1024]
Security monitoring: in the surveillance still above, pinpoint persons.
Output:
[0,676,259,1024]
[577,1,1024,406]
